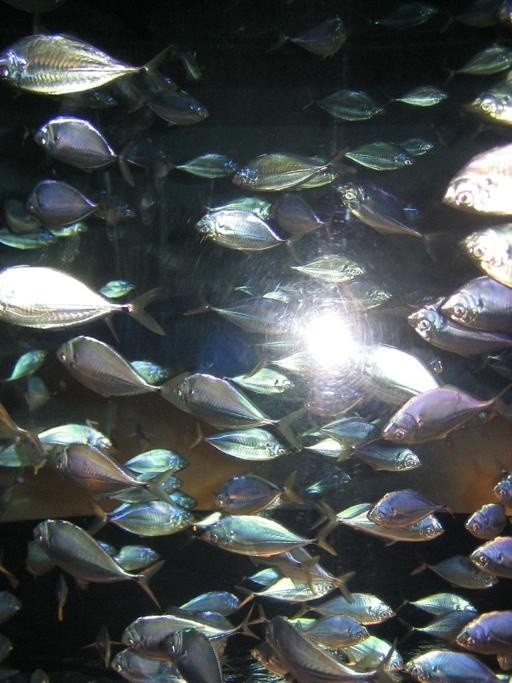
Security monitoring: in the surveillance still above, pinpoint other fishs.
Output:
[0,0,512,683]
